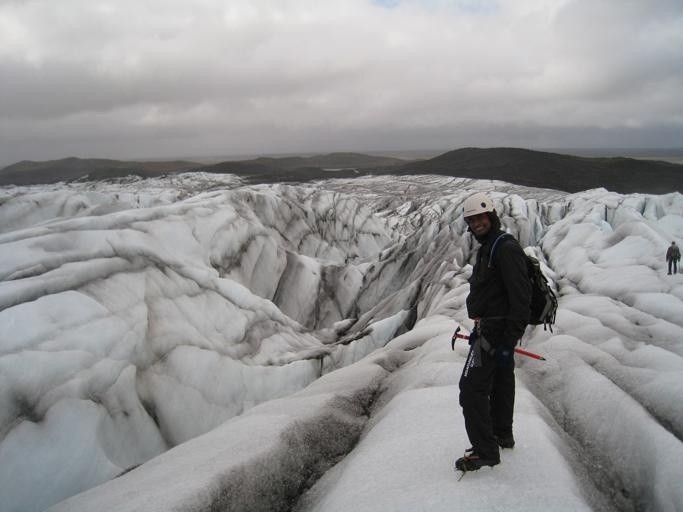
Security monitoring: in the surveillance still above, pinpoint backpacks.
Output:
[487,231,558,326]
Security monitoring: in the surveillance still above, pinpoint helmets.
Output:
[462,192,493,219]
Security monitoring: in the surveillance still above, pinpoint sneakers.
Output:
[454,451,501,472]
[493,434,515,449]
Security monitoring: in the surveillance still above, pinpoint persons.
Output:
[454,191,534,472]
[664,240,681,276]
